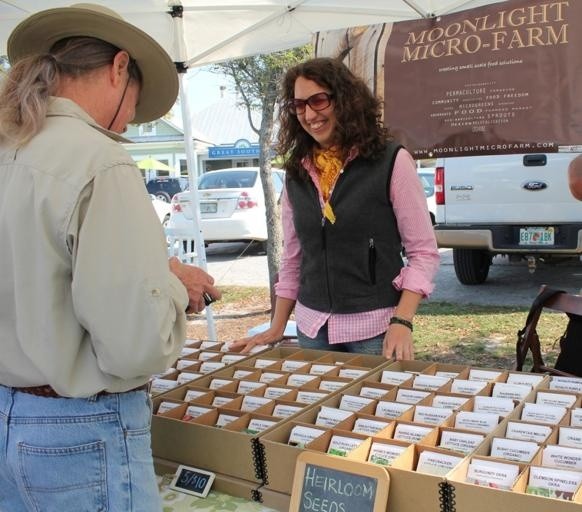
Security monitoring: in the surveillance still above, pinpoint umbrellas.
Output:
[134,153,181,187]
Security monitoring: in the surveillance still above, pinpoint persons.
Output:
[223,56,442,362]
[1,2,225,510]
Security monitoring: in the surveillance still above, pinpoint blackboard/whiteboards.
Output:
[168,464,217,498]
[289,450,390,512]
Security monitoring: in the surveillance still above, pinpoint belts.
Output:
[11,383,148,399]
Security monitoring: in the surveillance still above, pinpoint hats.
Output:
[7,3,179,124]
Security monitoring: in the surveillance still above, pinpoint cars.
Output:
[413,166,439,228]
[147,194,173,234]
[162,164,287,258]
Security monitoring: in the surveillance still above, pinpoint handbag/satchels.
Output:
[516,287,582,377]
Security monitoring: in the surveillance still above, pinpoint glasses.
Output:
[286,92,336,115]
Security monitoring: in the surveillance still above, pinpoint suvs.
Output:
[424,137,581,289]
[145,175,191,204]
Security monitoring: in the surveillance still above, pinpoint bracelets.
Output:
[387,315,414,333]
